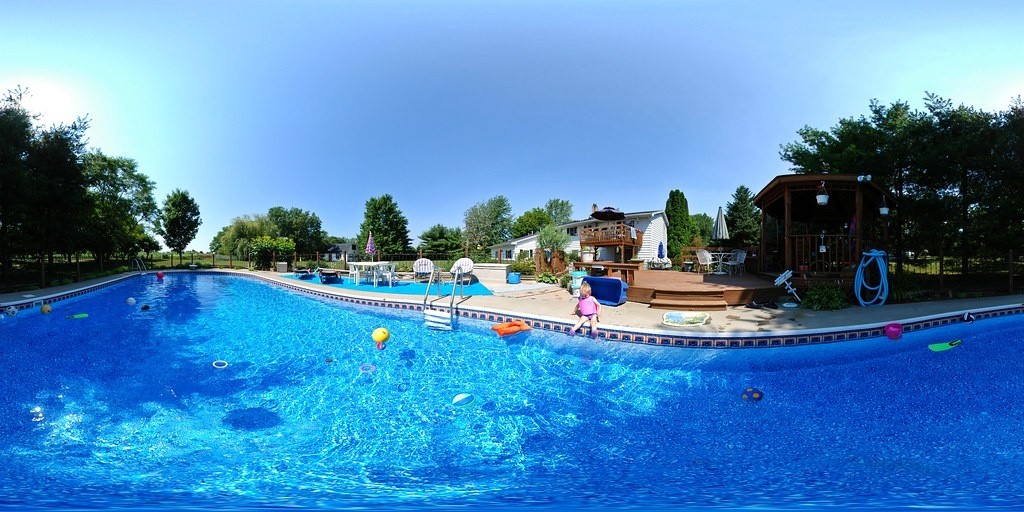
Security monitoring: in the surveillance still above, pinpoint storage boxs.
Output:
[570,271,587,296]
[507,272,521,284]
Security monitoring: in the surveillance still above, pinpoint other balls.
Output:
[156,272,163,278]
[371,328,390,342]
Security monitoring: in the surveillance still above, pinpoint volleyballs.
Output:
[41,304,52,315]
[126,297,136,306]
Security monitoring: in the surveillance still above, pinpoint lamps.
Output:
[857,174,872,184]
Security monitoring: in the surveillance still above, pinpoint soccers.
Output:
[5,306,19,318]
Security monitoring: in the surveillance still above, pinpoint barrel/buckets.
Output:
[570,271,587,284]
[571,284,582,296]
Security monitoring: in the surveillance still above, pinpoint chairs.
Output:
[695,248,748,278]
[349,263,396,289]
[412,258,437,284]
[293,267,342,284]
[448,258,474,287]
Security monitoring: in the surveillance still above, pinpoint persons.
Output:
[567,281,602,339]
[650,257,655,269]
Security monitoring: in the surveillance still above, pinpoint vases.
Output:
[879,207,890,215]
[816,194,830,206]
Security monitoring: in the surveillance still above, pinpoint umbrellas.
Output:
[658,241,665,261]
[712,207,730,253]
[365,231,376,262]
[590,207,626,225]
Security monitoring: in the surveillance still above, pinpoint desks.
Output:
[347,261,389,287]
[710,253,735,275]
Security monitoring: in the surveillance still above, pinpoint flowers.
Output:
[815,180,833,192]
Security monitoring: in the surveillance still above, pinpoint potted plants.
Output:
[683,257,694,272]
[275,254,288,273]
